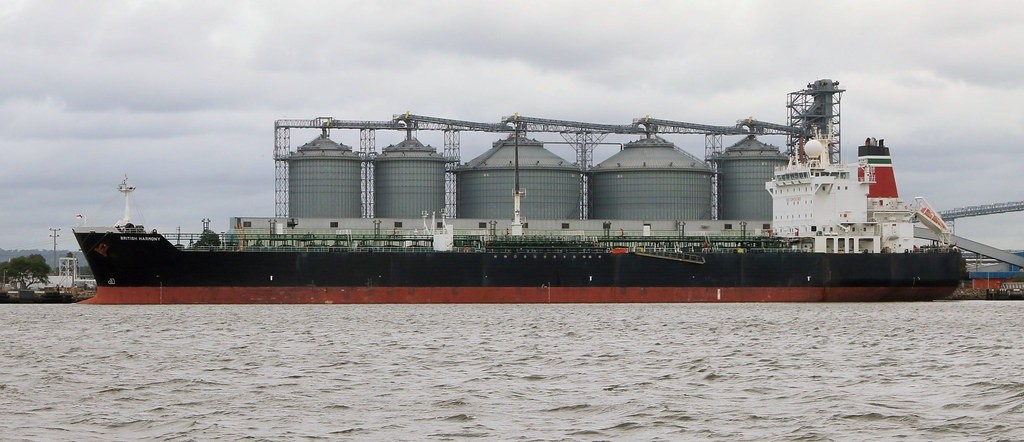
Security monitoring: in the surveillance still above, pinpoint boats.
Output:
[71,79,969,307]
